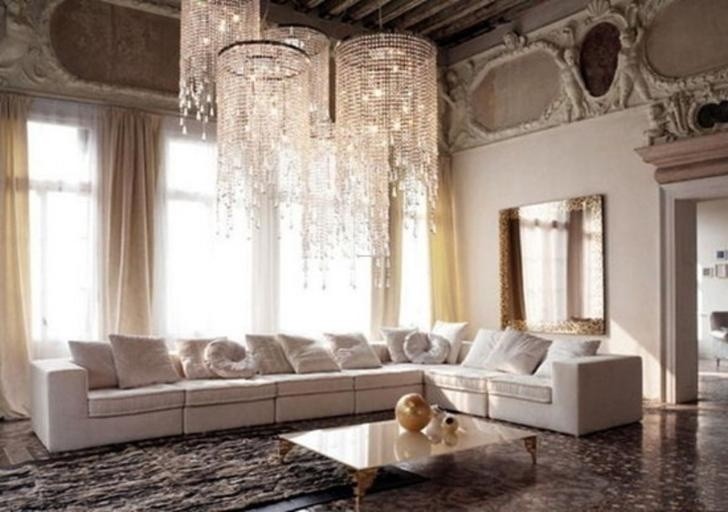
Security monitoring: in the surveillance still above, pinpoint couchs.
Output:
[26,329,644,453]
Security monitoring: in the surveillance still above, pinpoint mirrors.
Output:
[497,191,609,337]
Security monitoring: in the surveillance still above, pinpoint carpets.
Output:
[1,399,432,511]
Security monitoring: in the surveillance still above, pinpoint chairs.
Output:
[709,311,728,368]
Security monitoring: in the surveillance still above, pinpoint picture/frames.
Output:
[715,262,728,278]
